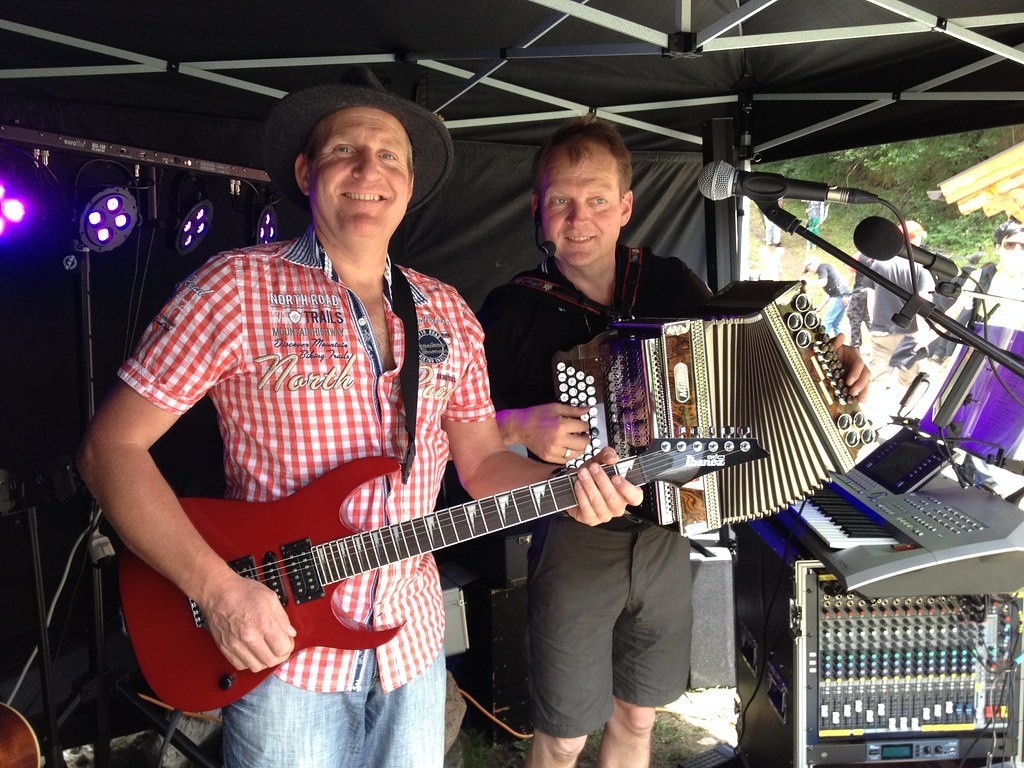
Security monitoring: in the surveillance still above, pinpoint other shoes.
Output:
[775,243,781,247]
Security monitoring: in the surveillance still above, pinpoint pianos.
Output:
[761,423,1024,601]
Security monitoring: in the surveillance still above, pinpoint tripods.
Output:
[35,195,216,768]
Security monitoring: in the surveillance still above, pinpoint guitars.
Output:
[116,424,771,712]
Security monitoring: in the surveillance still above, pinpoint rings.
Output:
[563,448,572,461]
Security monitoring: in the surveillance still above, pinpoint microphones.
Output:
[534,207,557,258]
[852,216,971,284]
[698,160,879,205]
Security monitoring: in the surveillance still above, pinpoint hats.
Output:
[803,256,818,275]
[258,64,454,215]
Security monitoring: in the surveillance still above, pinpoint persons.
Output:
[478,117,872,767]
[77,66,644,768]
[747,171,1024,424]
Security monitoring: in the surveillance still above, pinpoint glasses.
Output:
[1004,242,1024,251]
